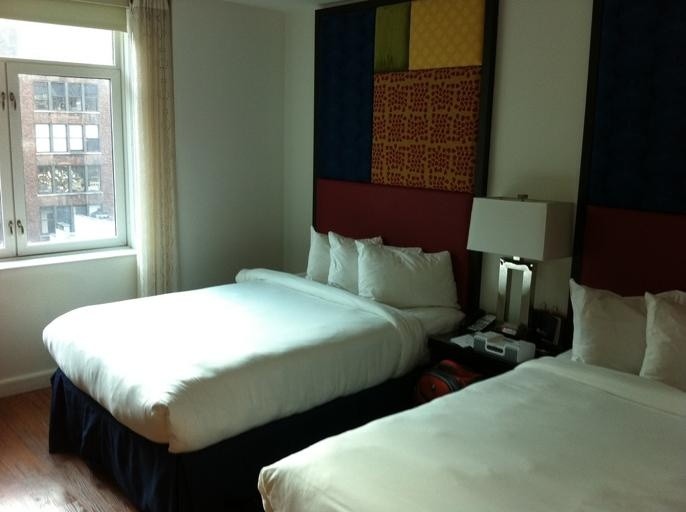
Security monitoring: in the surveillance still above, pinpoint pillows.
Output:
[566,277,686,388]
[299,226,465,316]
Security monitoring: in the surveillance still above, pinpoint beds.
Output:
[256,349,686,510]
[24,257,467,473]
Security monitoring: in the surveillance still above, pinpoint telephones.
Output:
[458,307,497,333]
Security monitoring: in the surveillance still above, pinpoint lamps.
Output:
[466,189,574,335]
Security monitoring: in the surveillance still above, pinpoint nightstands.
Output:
[422,322,549,382]
